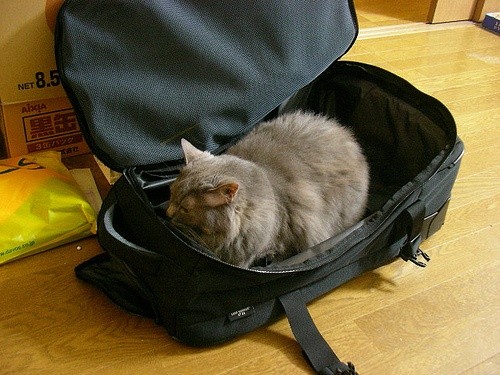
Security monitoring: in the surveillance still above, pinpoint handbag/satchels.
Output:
[54,0,465,375]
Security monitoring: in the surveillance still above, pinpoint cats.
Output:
[165,107,376,270]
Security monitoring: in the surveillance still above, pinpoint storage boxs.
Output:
[0,96,92,160]
